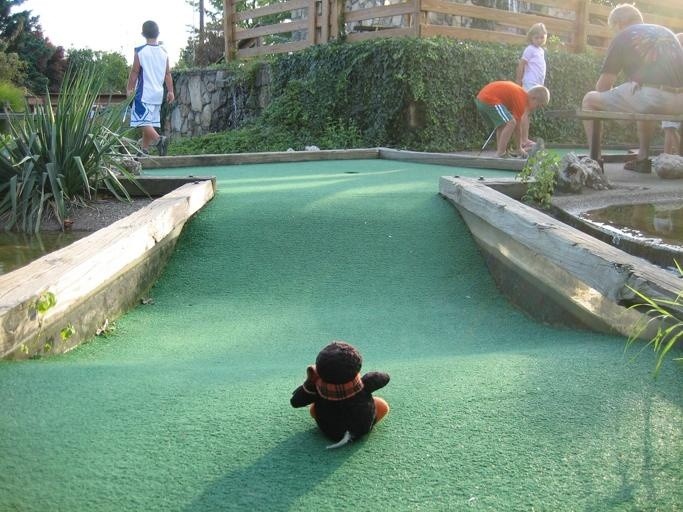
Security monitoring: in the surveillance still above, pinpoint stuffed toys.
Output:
[290,342,390,449]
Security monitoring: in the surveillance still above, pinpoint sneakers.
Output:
[624,158,653,174]
[498,143,528,160]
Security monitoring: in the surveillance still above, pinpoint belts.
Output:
[643,81,683,94]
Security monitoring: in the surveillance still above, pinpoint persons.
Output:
[125,20,175,155]
[476,80,550,158]
[579,4,683,177]
[516,22,548,152]
[650,31,683,166]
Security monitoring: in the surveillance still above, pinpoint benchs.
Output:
[546,110,683,177]
